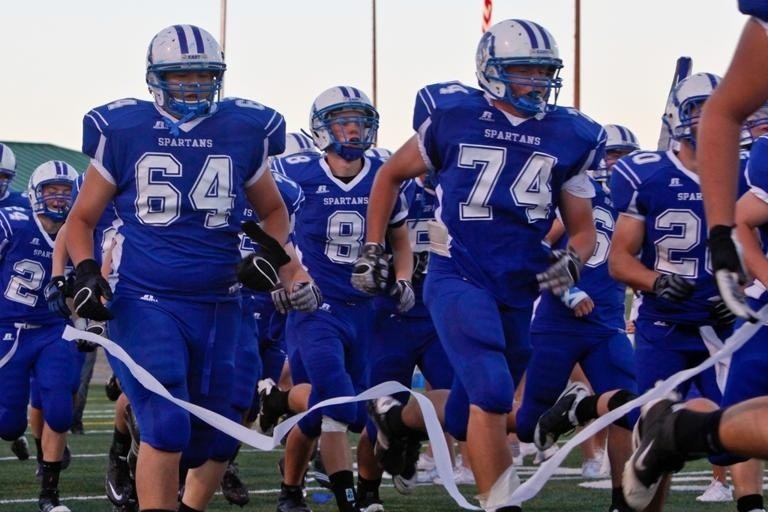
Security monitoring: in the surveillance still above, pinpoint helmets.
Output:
[662,73,723,150]
[738,104,768,159]
[147,25,227,137]
[310,86,379,161]
[28,160,79,222]
[585,124,641,181]
[476,20,564,115]
[0,144,16,200]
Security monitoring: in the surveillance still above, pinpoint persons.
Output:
[2,1,768,511]
